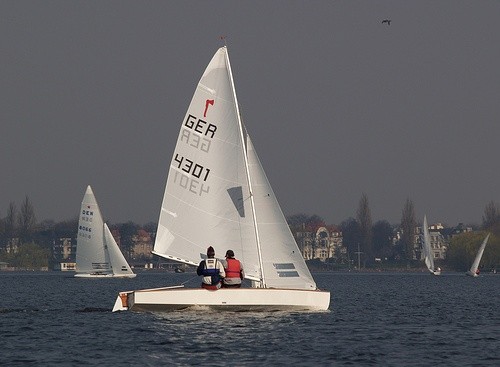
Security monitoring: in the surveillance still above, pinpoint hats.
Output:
[225,250,234,257]
[207,245,215,256]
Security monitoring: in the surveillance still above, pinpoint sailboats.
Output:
[72,185,138,279]
[421,215,441,276]
[111,37,331,313]
[465,232,491,277]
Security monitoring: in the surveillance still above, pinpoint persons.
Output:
[200,250,245,287]
[197,246,226,290]
